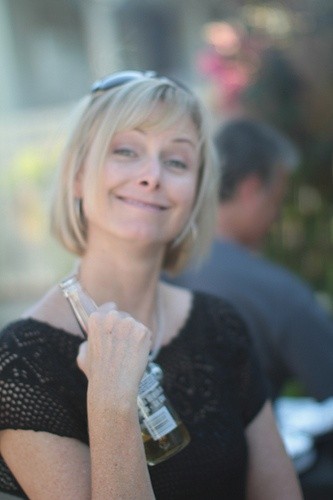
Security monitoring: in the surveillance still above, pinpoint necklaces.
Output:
[75,260,163,362]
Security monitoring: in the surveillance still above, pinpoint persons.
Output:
[223,26,312,153]
[160,108,333,500]
[0,69,302,500]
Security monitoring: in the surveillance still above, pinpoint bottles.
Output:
[59,275,190,466]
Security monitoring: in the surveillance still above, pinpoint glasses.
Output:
[92,70,194,104]
